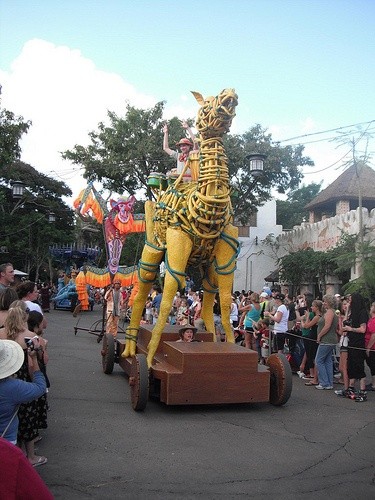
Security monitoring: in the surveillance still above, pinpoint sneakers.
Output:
[348,391,367,401]
[338,387,354,397]
[297,370,344,389]
[365,383,375,391]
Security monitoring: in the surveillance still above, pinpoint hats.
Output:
[259,287,285,300]
[1,339,23,379]
[178,324,198,337]
[335,294,341,297]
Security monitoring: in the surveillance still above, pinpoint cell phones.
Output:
[244,298,249,301]
[304,310,307,314]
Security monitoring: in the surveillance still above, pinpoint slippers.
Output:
[33,436,43,444]
[31,456,47,467]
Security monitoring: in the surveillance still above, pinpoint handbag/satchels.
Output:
[343,318,353,332]
[232,321,239,328]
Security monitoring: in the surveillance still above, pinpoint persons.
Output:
[304,300,324,386]
[0,260,81,311]
[24,310,48,443]
[334,298,354,395]
[161,119,198,181]
[0,336,47,447]
[315,294,338,390]
[0,287,18,328]
[0,300,48,469]
[15,281,47,335]
[338,293,368,402]
[86,278,353,384]
[365,301,375,391]
[1,436,55,500]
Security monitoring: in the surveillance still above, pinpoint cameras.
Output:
[343,320,350,332]
[24,336,39,348]
